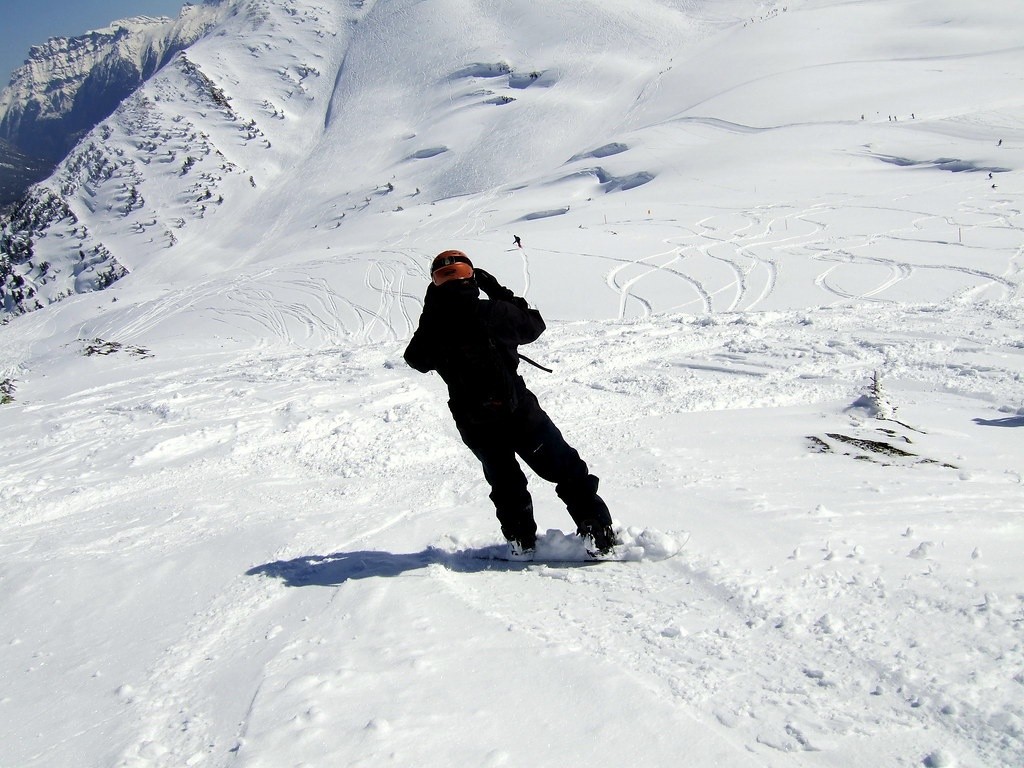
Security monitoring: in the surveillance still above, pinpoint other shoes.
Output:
[509,537,535,559]
[585,527,616,557]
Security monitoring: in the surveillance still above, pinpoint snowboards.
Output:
[468,527,690,563]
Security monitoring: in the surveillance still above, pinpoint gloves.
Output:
[473,265,500,300]
[423,282,442,315]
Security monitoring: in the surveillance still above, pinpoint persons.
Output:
[403,250,617,561]
[513,235,521,248]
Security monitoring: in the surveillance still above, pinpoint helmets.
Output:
[430,249,475,290]
